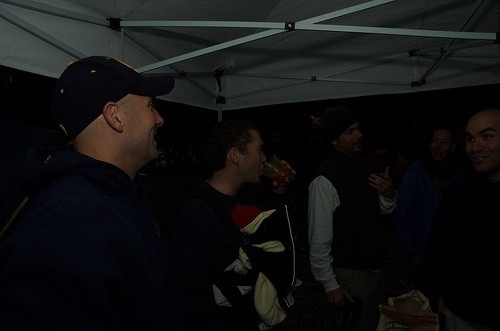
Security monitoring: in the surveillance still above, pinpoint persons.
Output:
[307,109,398,331]
[0,55,182,331]
[466,106,496,331]
[402,123,473,331]
[175,118,303,331]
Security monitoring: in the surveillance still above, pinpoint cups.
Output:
[261,155,295,187]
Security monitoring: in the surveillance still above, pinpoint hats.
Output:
[53,56,175,140]
[324,108,356,145]
